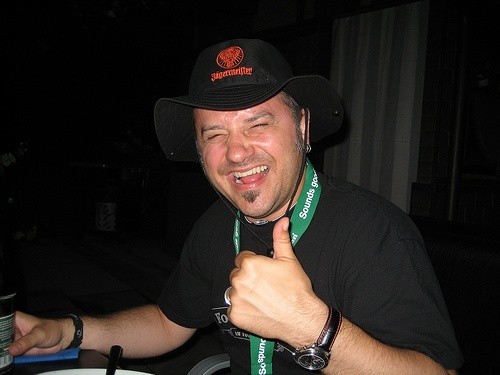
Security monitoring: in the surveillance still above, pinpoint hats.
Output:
[154,38,345,163]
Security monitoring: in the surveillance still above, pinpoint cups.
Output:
[95,202,116,231]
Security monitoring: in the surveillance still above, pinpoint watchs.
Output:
[294,305,342,371]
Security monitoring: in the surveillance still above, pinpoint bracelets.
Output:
[66,314,83,350]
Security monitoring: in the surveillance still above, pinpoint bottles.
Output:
[0,252,14,374]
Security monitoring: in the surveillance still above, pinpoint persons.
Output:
[8,39,461,375]
